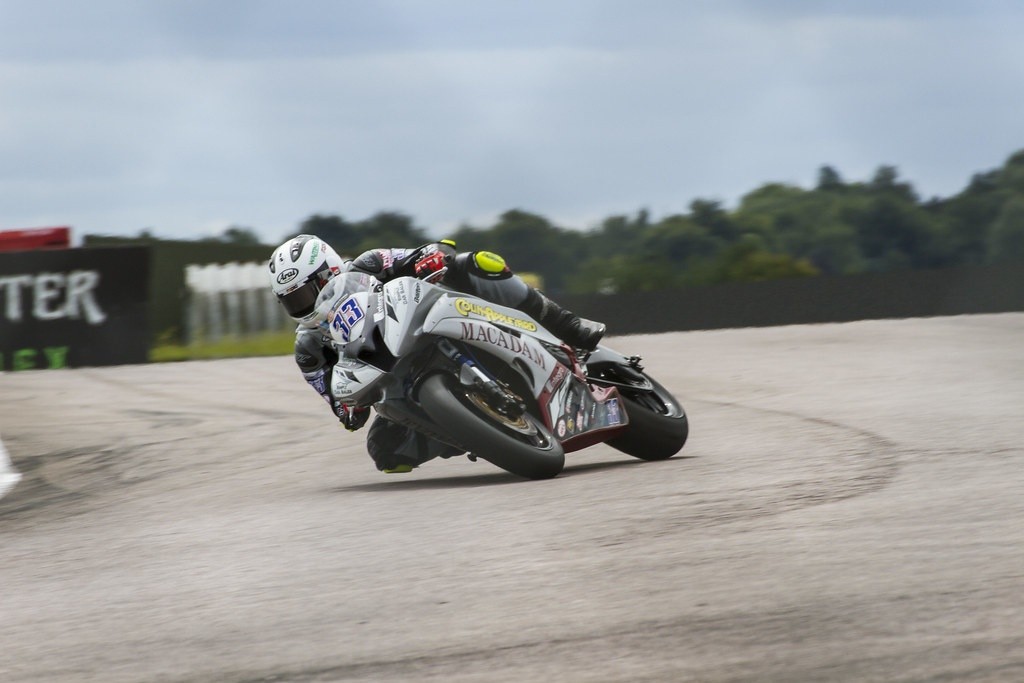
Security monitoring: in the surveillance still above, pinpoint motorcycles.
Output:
[314,250,690,481]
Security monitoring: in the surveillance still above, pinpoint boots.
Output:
[513,282,607,351]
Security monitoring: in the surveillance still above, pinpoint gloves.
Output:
[343,412,370,431]
[414,250,445,279]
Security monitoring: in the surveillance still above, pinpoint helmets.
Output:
[268,233,347,329]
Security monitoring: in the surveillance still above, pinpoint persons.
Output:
[266,233,608,474]
